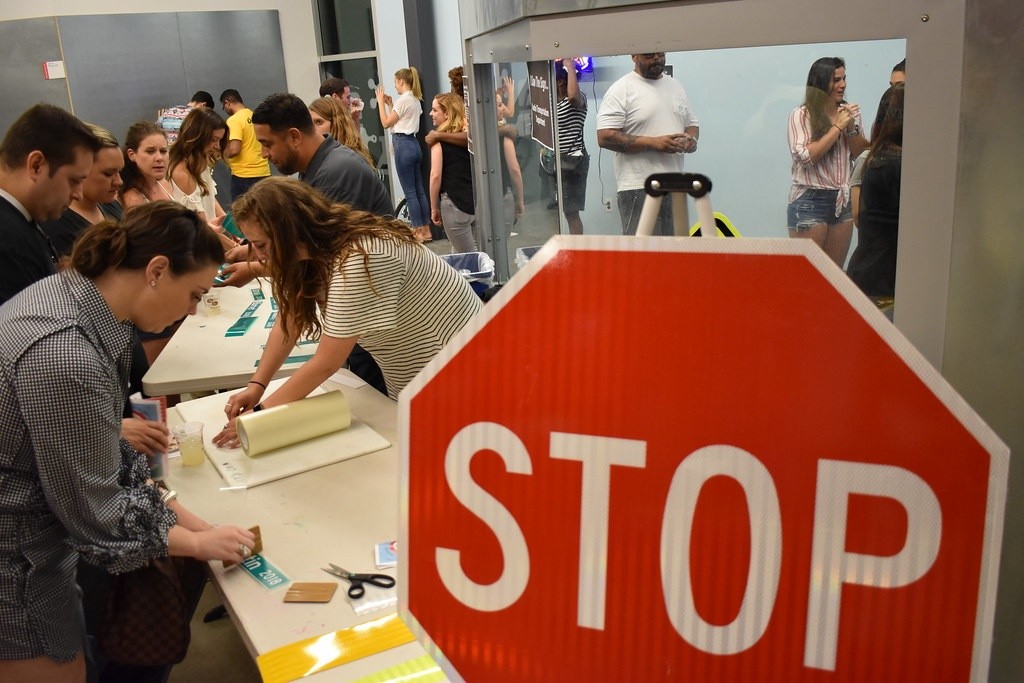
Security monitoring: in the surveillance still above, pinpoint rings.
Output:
[226,404,232,407]
[239,545,249,557]
[224,423,228,429]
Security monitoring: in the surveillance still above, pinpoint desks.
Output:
[141,276,347,397]
[161,367,452,683]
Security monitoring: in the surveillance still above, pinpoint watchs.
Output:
[847,125,860,136]
[253,403,262,412]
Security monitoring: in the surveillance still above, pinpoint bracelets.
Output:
[834,124,842,132]
[162,490,176,506]
[249,381,266,389]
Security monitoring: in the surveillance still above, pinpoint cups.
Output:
[349,96,365,111]
[202,288,222,314]
[171,421,203,466]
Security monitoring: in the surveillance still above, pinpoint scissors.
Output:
[321,563,396,599]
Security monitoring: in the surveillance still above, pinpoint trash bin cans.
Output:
[439,251,493,297]
[516,246,542,269]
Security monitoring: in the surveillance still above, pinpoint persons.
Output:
[0,77,387,455]
[788,56,868,270]
[426,67,476,255]
[0,200,256,683]
[539,58,589,235]
[846,58,906,324]
[211,175,484,450]
[596,52,699,235]
[495,76,524,243]
[375,66,432,244]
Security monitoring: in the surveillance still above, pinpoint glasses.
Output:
[639,52,667,58]
[221,103,226,111]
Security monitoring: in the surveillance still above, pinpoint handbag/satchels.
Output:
[94,562,191,666]
[554,149,590,177]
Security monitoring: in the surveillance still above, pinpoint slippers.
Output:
[411,232,433,243]
